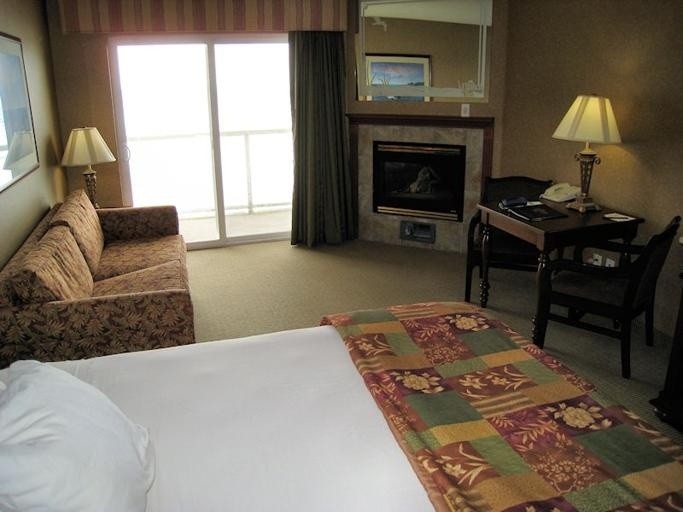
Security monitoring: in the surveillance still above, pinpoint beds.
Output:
[0,300,682,511]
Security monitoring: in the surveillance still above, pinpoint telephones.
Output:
[539,181,581,202]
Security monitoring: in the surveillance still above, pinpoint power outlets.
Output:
[592,252,603,267]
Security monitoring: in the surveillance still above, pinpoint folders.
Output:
[507,203,570,223]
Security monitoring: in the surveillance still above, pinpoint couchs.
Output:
[0,187,192,359]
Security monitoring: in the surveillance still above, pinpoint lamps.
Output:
[59,125,119,210]
[550,92,620,211]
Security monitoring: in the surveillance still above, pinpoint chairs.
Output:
[534,209,681,375]
[464,176,564,313]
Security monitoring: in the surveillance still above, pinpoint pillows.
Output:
[0,362,150,512]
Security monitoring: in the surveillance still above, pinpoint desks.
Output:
[474,197,646,358]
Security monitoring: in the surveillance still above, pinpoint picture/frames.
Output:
[356,51,433,102]
[1,28,42,195]
